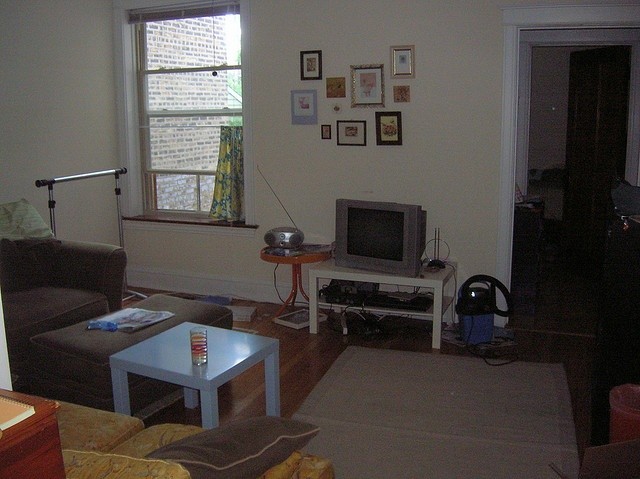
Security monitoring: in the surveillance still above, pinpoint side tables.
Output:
[261,245,332,321]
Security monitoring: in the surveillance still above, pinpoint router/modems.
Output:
[428,227,446,270]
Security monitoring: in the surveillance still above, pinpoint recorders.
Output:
[263,226,305,249]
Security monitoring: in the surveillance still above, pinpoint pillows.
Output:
[0,199,53,241]
[144,415,321,479]
[0,238,61,291]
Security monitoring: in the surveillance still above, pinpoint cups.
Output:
[190,326,208,365]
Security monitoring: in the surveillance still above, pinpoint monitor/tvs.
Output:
[335,197,427,277]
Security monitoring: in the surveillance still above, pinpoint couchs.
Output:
[43,399,336,479]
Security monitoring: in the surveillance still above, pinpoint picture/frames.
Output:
[350,64,386,108]
[321,125,331,140]
[299,50,322,80]
[276,309,328,330]
[337,119,367,147]
[375,111,402,146]
[390,45,416,80]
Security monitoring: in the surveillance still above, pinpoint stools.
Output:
[29,294,234,419]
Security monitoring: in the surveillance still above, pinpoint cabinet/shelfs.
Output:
[0,387,65,478]
[309,258,457,349]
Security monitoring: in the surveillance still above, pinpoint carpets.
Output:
[292,346,579,478]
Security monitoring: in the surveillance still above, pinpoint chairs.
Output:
[0,236,126,358]
